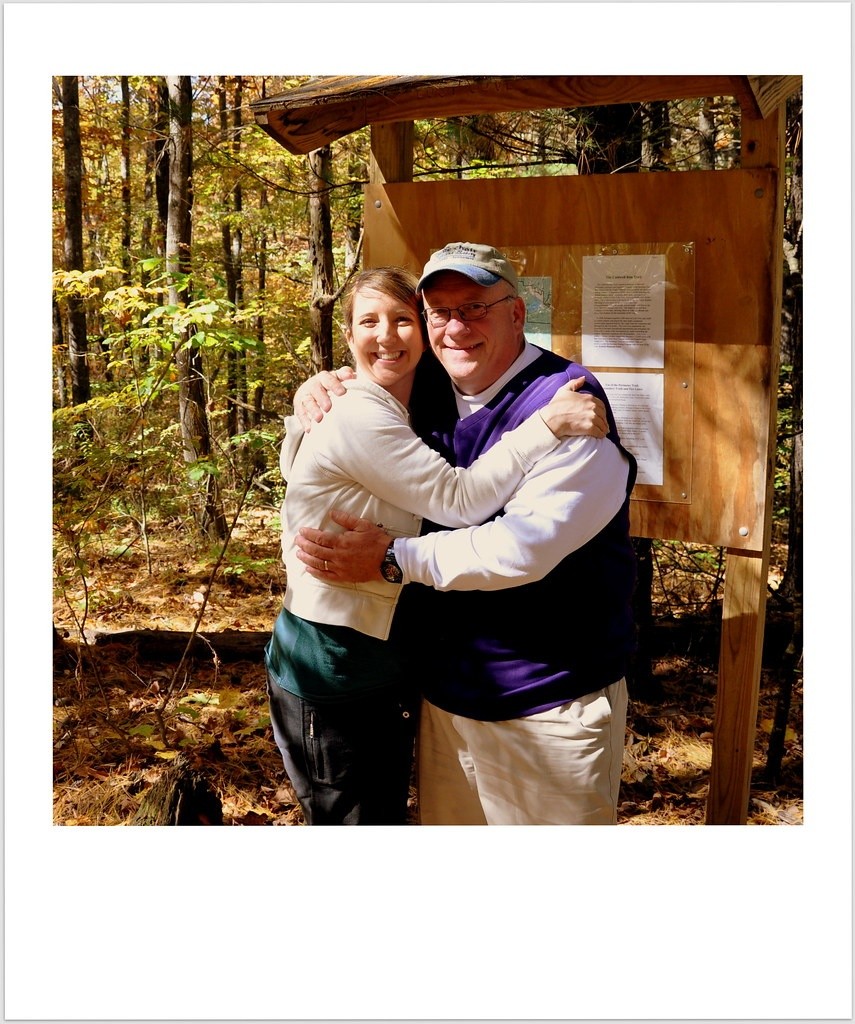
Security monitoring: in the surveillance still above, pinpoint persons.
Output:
[278,243,654,825]
[263,264,612,825]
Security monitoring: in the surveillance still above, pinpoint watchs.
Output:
[379,536,404,584]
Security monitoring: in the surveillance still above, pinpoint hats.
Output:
[415,242,516,294]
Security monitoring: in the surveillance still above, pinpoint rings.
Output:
[324,560,329,571]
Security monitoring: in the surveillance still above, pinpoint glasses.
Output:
[420,296,512,322]
